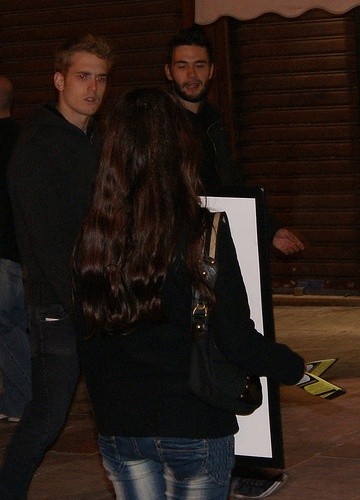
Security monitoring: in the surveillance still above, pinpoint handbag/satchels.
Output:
[190,211,263,416]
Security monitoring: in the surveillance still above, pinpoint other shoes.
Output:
[0,414,20,422]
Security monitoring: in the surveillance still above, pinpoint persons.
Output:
[162,31,302,255]
[67,84,304,500]
[0,32,109,500]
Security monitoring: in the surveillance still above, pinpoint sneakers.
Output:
[229,472,288,499]
